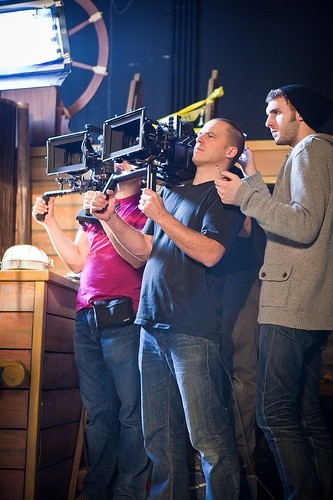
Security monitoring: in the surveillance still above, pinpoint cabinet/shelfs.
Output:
[0,269,80,499]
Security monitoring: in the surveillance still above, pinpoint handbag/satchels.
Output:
[89,296,135,329]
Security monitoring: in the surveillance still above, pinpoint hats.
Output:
[281,85,331,130]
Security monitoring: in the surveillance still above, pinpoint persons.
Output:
[90,117,254,500]
[32,160,156,500]
[215,87,333,500]
[180,164,264,500]
